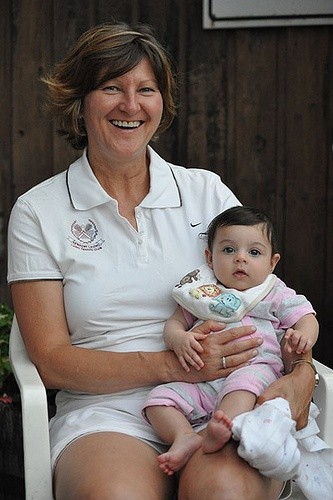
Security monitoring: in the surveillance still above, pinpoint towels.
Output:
[229,396,333,500]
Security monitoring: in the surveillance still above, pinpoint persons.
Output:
[8,22,315,500]
[143,205,319,475]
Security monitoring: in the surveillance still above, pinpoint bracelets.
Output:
[291,359,319,388]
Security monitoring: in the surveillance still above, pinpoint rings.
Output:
[223,358,226,369]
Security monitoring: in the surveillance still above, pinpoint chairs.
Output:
[8,309,333,500]
